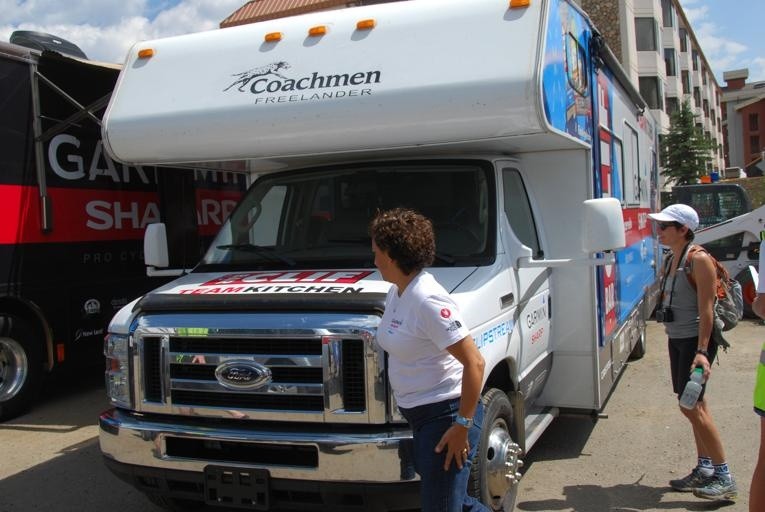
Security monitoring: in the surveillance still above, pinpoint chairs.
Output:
[290,207,473,258]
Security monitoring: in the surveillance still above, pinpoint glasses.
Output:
[659,223,678,231]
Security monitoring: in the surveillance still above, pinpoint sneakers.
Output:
[692,473,738,500]
[668,467,713,492]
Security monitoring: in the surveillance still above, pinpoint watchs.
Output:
[456,416,474,429]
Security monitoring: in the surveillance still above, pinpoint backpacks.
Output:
[661,245,744,332]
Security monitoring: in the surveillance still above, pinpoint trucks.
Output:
[98,0,665,512]
[0,39,253,426]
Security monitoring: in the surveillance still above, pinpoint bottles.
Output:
[679,368,707,410]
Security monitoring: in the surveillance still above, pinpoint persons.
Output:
[367,207,491,512]
[647,203,735,503]
[749,239,765,511]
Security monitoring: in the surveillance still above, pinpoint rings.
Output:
[461,449,467,455]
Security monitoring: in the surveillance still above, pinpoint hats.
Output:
[646,204,700,232]
